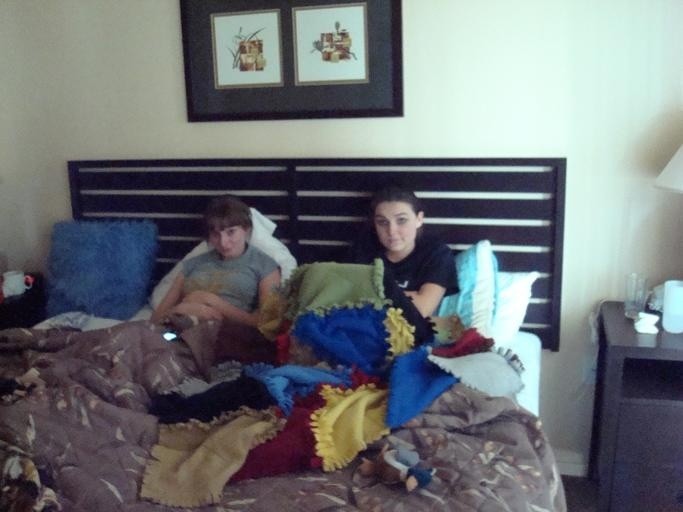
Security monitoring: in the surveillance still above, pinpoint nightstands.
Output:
[590,296,682,512]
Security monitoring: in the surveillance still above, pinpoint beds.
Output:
[1,154,572,511]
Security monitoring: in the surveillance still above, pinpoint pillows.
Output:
[488,270,542,350]
[49,215,158,319]
[301,237,498,343]
[147,206,291,328]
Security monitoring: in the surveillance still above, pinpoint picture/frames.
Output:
[177,0,406,124]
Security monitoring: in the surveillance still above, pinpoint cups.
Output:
[661,278,682,334]
[4,269,32,295]
[622,272,648,318]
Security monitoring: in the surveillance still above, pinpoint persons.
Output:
[324,188,458,325]
[151,192,282,342]
